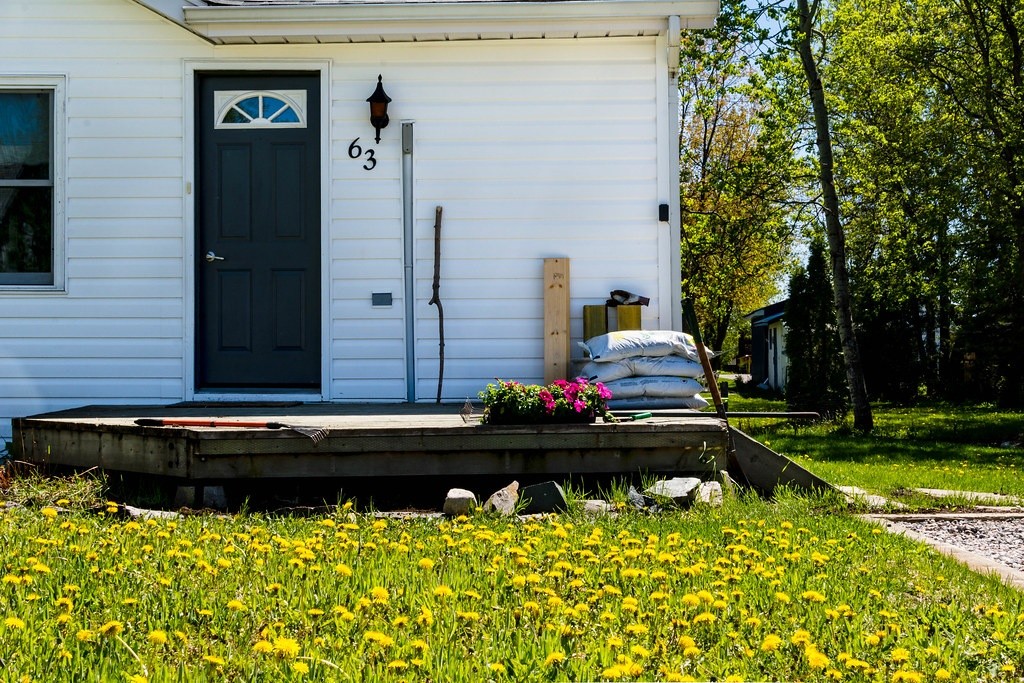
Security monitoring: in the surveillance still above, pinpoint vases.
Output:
[487,402,596,426]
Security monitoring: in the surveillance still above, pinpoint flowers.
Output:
[475,374,619,423]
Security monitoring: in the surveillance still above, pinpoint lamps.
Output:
[365,72,392,144]
